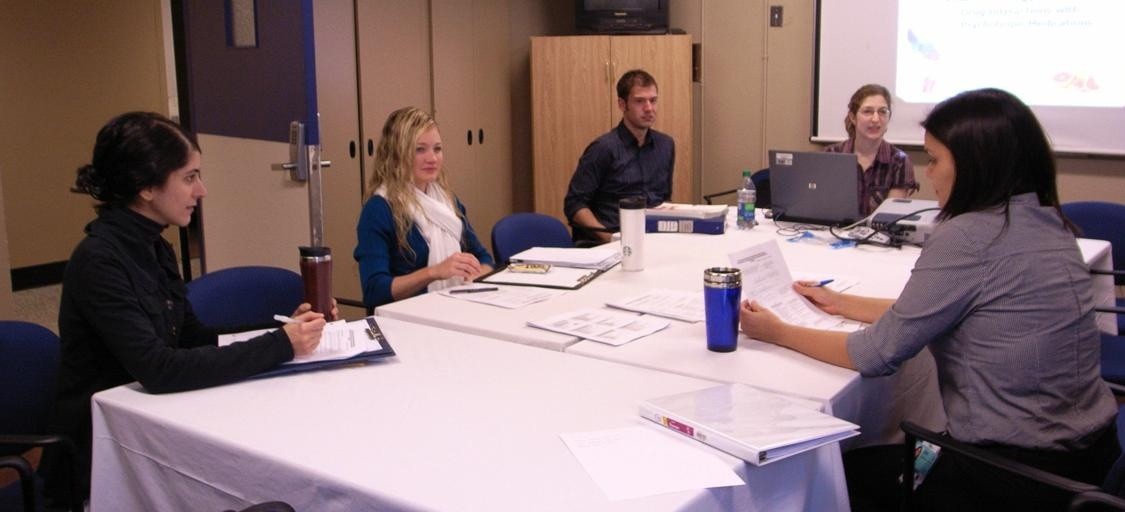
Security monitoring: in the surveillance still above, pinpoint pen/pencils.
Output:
[815,280,833,287]
[273,314,305,324]
[450,288,498,293]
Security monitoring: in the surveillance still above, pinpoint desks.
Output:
[374,203,1115,476]
[88,309,857,512]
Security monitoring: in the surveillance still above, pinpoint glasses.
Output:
[858,105,891,117]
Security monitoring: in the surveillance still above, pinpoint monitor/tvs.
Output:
[575,0,668,35]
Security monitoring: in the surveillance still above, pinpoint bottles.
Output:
[735,169,758,229]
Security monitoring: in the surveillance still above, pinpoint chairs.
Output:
[1,317,86,511]
[181,266,315,330]
[898,377,1124,512]
[1053,197,1124,277]
[1086,300,1125,388]
[704,165,772,210]
[488,210,574,269]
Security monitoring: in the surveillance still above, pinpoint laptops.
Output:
[768,150,859,225]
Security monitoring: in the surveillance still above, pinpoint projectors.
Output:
[867,198,939,244]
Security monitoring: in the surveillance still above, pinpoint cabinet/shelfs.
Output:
[426,1,512,262]
[311,0,435,307]
[666,0,818,207]
[529,33,695,225]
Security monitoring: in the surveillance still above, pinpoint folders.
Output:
[646,215,725,234]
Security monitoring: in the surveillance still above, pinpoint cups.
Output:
[703,265,742,352]
[618,197,646,270]
[297,245,334,323]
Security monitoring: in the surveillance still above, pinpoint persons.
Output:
[739,90,1118,512]
[358,107,496,316]
[565,70,675,247]
[816,85,915,219]
[39,111,340,508]
